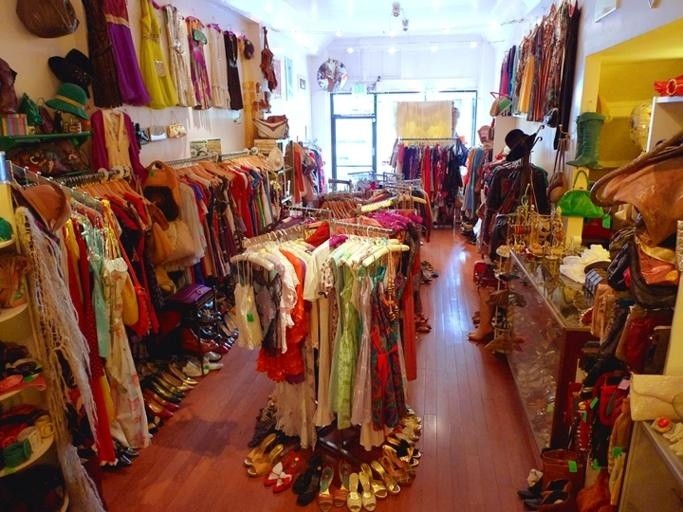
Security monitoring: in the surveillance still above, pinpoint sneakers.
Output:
[517,448,582,512]
[468,285,496,344]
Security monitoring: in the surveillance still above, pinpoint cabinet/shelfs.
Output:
[501,246,671,469]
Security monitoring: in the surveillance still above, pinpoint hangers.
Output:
[228,192,411,273]
[70,174,139,202]
[168,152,272,187]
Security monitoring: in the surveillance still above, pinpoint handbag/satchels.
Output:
[253,115,290,139]
[546,141,610,223]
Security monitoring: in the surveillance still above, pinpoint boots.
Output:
[566,111,606,169]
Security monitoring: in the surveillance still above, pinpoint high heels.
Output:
[417,260,441,287]
[72,308,242,472]
[244,389,426,512]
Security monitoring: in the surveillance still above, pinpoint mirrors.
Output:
[316,59,348,92]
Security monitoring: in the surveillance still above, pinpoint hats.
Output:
[502,127,536,161]
[42,80,92,123]
[47,47,98,100]
[8,180,72,236]
[265,146,286,173]
